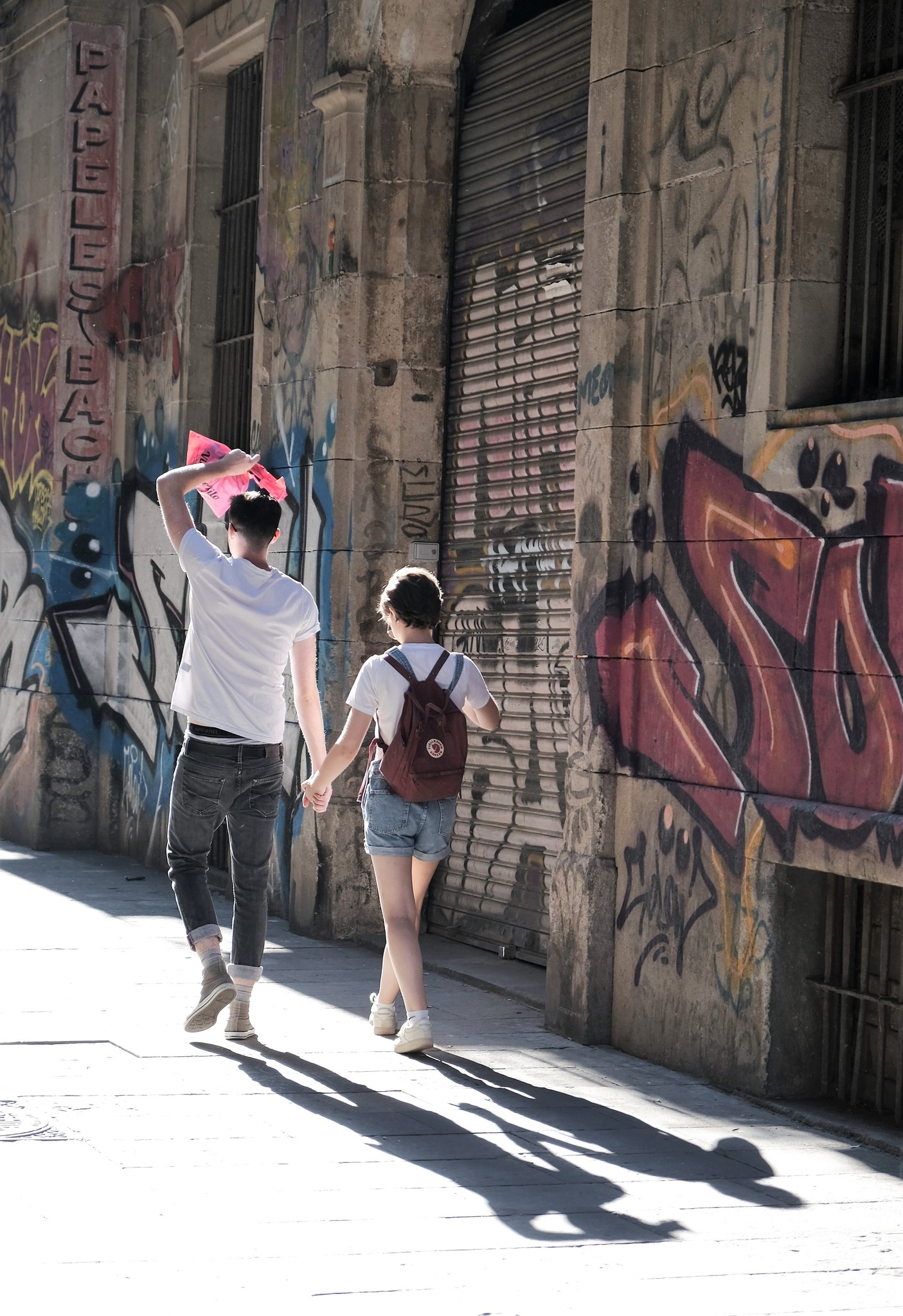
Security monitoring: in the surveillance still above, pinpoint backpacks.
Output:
[356,650,469,802]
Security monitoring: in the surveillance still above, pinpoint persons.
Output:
[301,565,501,1053]
[155,448,333,1040]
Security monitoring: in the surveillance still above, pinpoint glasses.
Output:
[224,520,237,533]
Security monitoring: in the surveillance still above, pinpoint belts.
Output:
[183,739,284,758]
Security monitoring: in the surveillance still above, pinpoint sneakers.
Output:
[369,992,397,1035]
[393,1019,434,1053]
[182,958,237,1033]
[224,999,254,1039]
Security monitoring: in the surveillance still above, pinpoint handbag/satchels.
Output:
[186,430,288,518]
